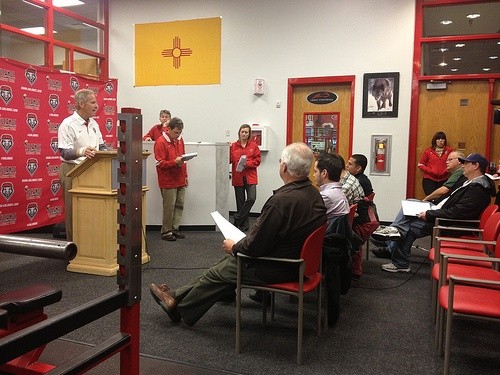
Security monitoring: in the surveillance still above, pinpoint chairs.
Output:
[234,192,375,366]
[430,205,500,375]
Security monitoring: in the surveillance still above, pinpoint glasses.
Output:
[446,157,457,160]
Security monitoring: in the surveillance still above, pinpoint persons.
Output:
[229,124,262,232]
[346,154,373,196]
[154,116,189,241]
[150,144,329,328]
[57,88,117,242]
[371,153,497,274]
[369,150,468,258]
[334,155,365,223]
[416,131,456,205]
[248,150,350,306]
[141,109,172,143]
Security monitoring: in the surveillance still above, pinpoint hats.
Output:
[458,154,488,169]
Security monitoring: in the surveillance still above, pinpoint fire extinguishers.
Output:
[375,139,387,171]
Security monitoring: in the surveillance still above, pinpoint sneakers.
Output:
[372,247,392,258]
[149,283,183,322]
[370,238,389,247]
[249,291,271,305]
[371,225,401,241]
[382,262,411,273]
[233,214,249,232]
[161,232,185,241]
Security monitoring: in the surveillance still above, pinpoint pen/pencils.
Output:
[416,214,419,215]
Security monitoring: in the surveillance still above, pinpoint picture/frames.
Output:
[362,72,400,118]
